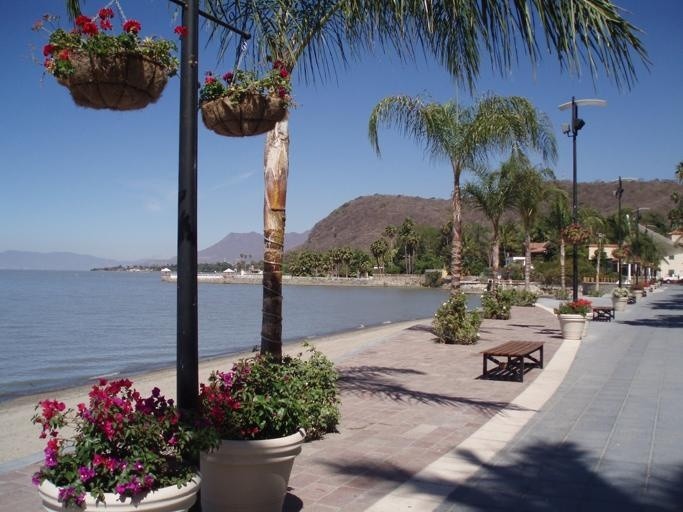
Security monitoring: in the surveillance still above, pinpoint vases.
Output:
[611,290,643,311]
[200,94,285,138]
[558,314,589,340]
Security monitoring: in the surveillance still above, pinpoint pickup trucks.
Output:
[663,274,683,284]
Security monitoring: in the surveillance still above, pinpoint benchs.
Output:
[593,306,615,322]
[480,342,546,381]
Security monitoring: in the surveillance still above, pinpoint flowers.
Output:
[30,6,293,108]
[200,339,342,443]
[612,280,650,297]
[32,376,200,506]
[554,299,592,316]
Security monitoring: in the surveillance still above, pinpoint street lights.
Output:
[557,96,607,304]
[611,176,657,289]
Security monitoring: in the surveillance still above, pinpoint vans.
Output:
[504,256,525,270]
